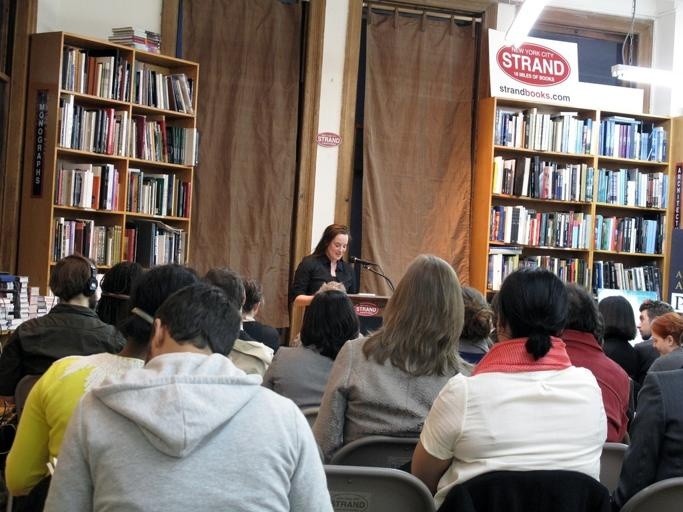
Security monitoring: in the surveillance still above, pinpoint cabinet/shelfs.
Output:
[15,31,201,298]
[469,97,683,334]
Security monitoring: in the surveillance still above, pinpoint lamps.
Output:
[610,0,668,86]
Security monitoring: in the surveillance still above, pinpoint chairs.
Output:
[618,477,683,512]
[301,406,320,431]
[15,376,40,423]
[442,470,613,511]
[328,437,435,478]
[600,443,628,498]
[324,465,435,511]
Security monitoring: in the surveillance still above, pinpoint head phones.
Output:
[66,255,98,297]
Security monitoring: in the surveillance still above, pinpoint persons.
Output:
[293,224,357,297]
[1,256,683,511]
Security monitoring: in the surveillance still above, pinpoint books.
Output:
[52,26,197,270]
[488,106,670,295]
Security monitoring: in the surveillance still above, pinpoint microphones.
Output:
[349,255,380,270]
